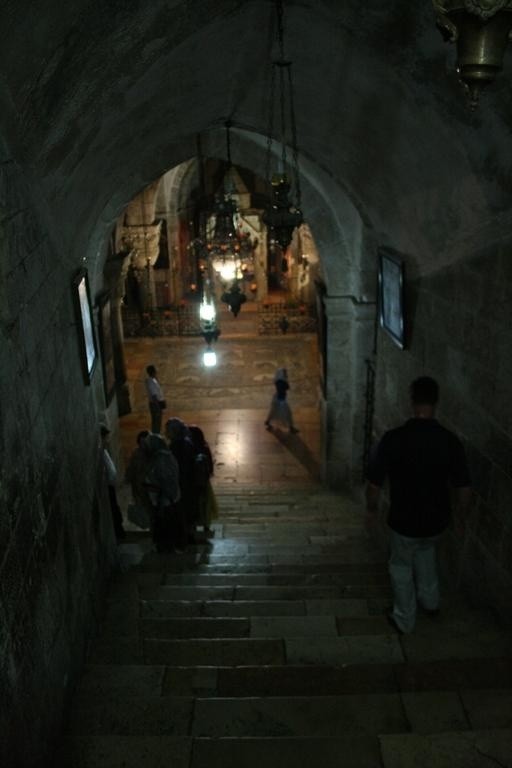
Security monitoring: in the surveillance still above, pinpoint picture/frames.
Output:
[373,246,407,351]
[71,265,98,387]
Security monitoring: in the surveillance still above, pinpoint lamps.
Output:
[429,1,512,114]
[183,0,305,316]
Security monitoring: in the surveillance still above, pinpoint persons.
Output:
[125,417,214,555]
[365,377,464,634]
[264,368,299,433]
[100,423,126,539]
[145,365,166,433]
